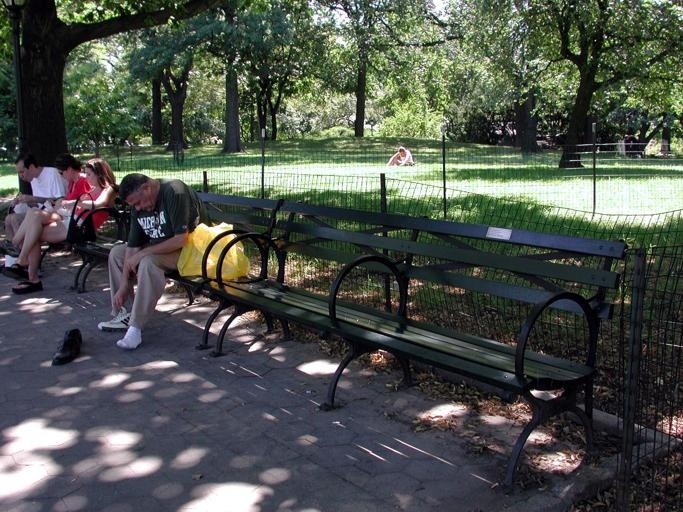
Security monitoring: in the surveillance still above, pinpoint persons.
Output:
[2,153,94,277]
[5,153,69,241]
[97,174,214,350]
[385,147,413,167]
[1,159,119,294]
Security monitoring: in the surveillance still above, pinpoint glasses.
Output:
[88,159,96,171]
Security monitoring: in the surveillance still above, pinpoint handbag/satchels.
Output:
[176,222,250,282]
[65,192,97,243]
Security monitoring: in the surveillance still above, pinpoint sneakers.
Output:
[53,328,83,366]
[97,306,132,331]
[0,239,21,258]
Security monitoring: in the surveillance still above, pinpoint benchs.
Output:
[36,186,124,290]
[204,187,627,496]
[77,186,280,351]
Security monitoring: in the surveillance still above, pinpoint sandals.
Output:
[12,280,43,295]
[2,263,29,280]
